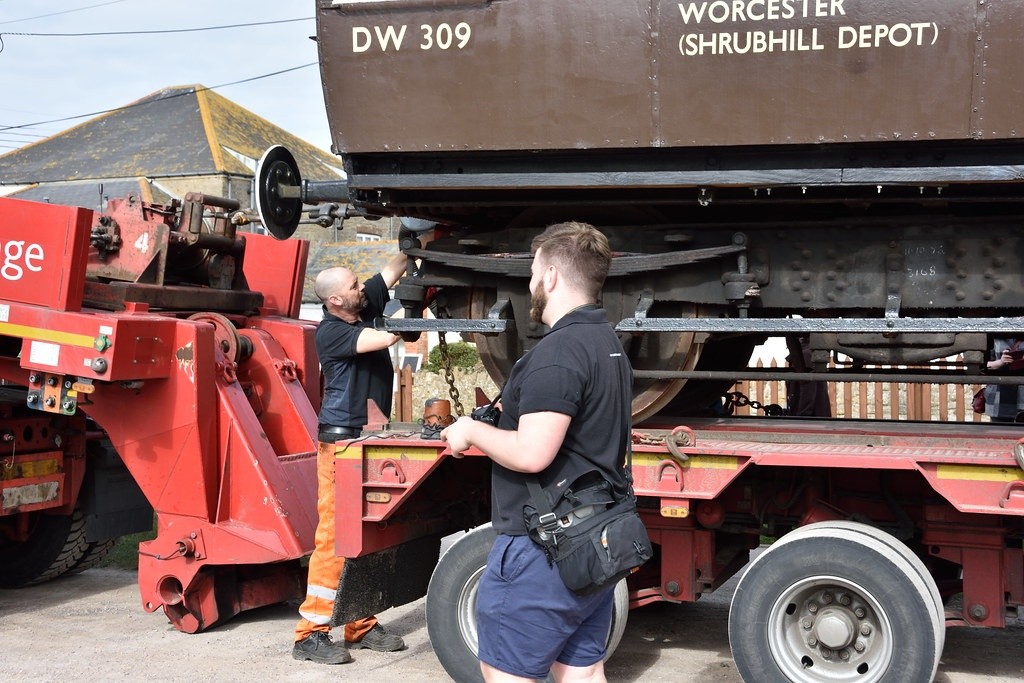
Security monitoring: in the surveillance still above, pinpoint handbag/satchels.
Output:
[523,469,655,593]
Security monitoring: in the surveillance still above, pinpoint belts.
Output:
[318,424,355,435]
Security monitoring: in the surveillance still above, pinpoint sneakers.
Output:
[344,621,404,652]
[292,632,352,664]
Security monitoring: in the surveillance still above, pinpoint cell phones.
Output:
[1005,349,1024,361]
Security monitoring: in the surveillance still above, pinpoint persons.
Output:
[289,228,445,666]
[983,334,1024,423]
[439,220,632,683]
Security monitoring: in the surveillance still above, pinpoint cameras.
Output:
[471,406,500,428]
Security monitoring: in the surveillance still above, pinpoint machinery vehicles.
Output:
[0,0,1022,683]
